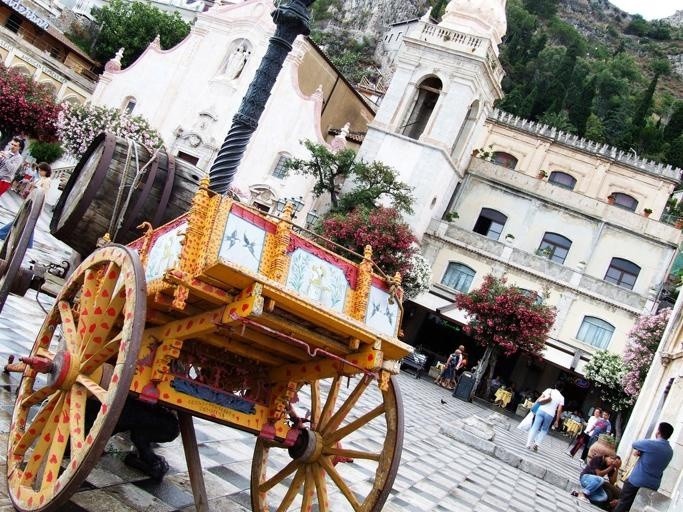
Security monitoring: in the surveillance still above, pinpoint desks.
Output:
[429,357,582,445]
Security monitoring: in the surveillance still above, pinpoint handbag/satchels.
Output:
[538,388,553,405]
[530,402,540,414]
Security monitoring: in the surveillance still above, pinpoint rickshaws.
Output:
[0,187,416,511]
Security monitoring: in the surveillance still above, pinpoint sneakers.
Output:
[570,490,590,504]
[527,445,537,452]
[564,452,584,463]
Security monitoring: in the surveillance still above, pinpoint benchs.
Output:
[399,350,428,380]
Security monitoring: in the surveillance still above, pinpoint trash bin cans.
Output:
[452,371,476,402]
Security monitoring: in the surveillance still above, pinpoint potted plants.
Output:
[537,168,549,180]
[643,208,652,219]
[674,216,682,229]
[586,430,615,466]
[575,258,586,271]
[607,195,617,205]
[505,231,515,244]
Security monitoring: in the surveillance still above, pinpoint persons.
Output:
[223,45,249,80]
[571,454,622,504]
[0,161,52,250]
[75,391,181,479]
[12,163,37,193]
[436,343,516,408]
[18,166,41,198]
[516,381,622,464]
[613,421,672,512]
[10,162,29,191]
[0,135,27,196]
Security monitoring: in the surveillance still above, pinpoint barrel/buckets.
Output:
[50,130,241,258]
